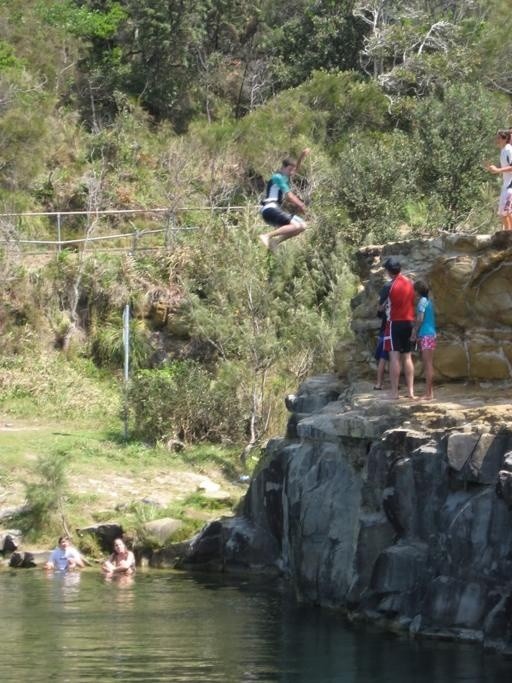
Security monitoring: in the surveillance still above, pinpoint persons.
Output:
[380,258,416,399]
[260,146,311,257]
[102,537,136,574]
[373,278,399,390]
[41,534,85,570]
[487,128,512,230]
[412,281,437,399]
